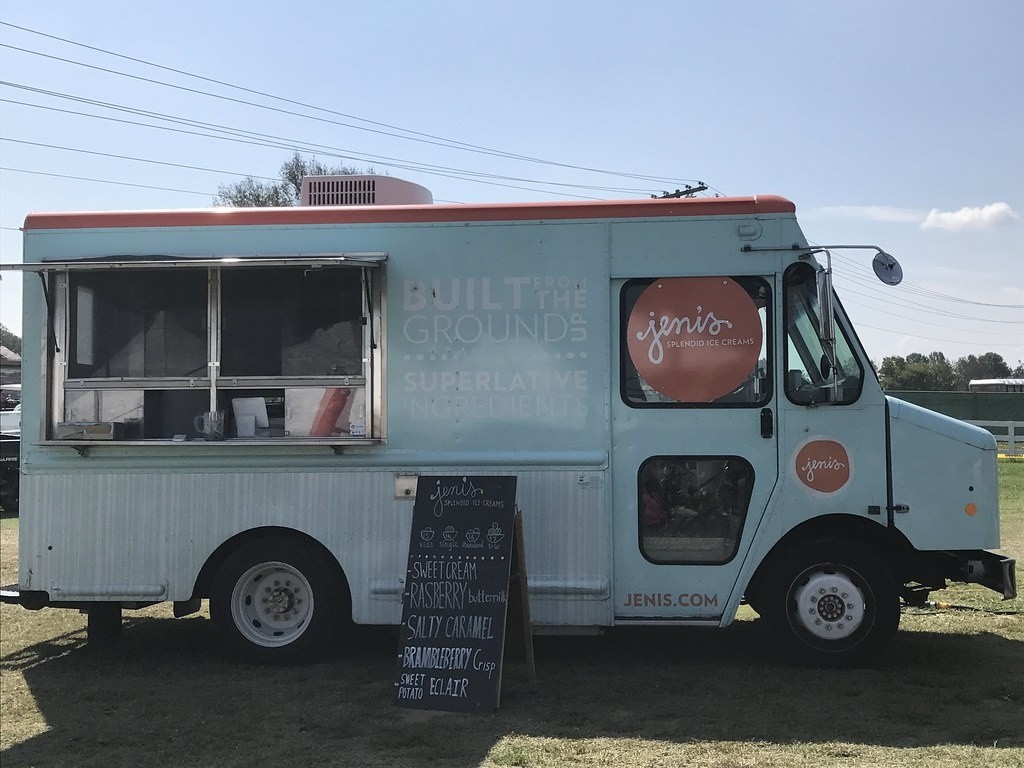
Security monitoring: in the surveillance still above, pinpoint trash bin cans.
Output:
[0,433,20,512]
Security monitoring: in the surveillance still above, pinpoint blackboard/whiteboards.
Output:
[389,475,540,712]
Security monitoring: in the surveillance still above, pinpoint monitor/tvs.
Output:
[232,397,268,437]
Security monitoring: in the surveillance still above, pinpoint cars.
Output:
[0,383,22,514]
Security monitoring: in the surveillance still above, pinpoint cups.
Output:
[194,411,224,441]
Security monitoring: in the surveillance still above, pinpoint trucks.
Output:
[0,170,1017,669]
